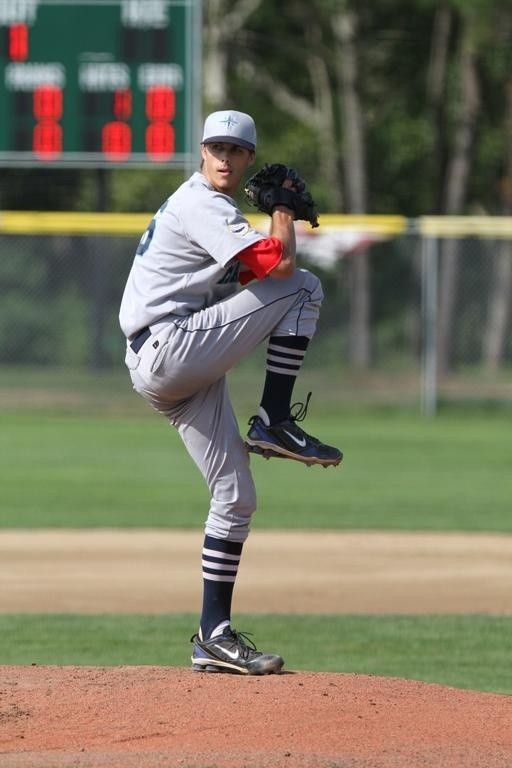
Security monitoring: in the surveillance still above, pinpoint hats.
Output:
[199,108,258,155]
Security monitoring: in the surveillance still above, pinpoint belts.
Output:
[130,326,153,356]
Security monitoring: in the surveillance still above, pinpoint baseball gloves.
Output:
[243,163,317,230]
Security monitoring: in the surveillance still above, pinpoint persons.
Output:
[118,109,345,677]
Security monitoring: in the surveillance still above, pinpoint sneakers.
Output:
[188,615,284,676]
[243,409,344,471]
